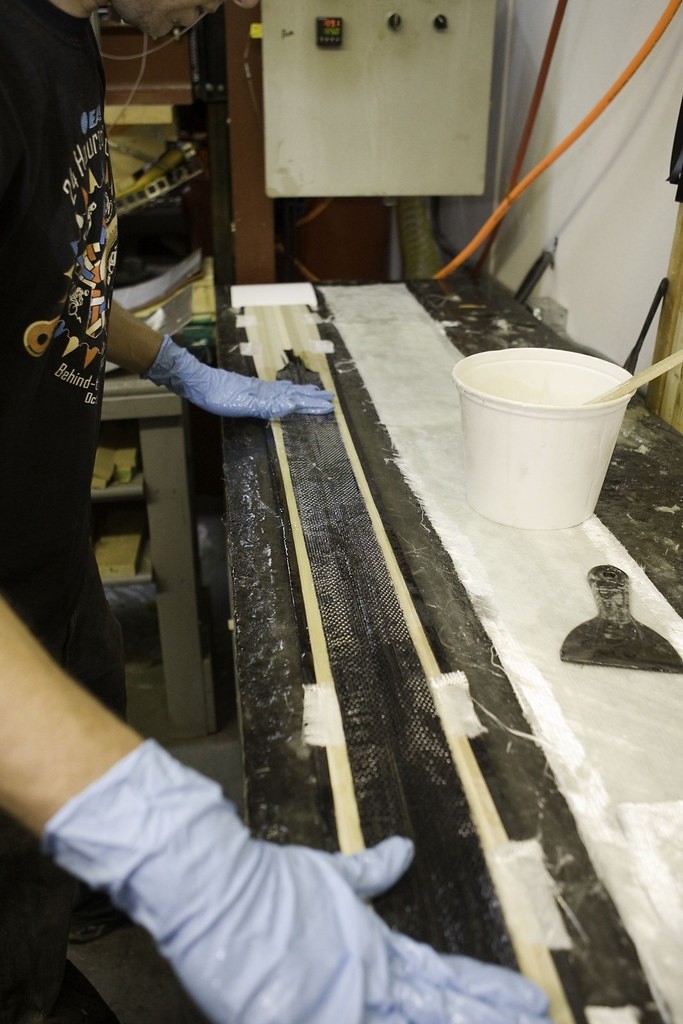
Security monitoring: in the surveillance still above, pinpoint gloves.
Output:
[139,334,334,418]
[39,737,552,1024]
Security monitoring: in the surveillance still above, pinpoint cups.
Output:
[452,349,636,529]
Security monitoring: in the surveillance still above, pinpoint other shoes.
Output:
[68,891,131,943]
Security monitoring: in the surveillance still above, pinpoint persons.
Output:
[0,0,553,1024]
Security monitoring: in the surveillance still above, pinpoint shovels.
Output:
[559,563,683,676]
